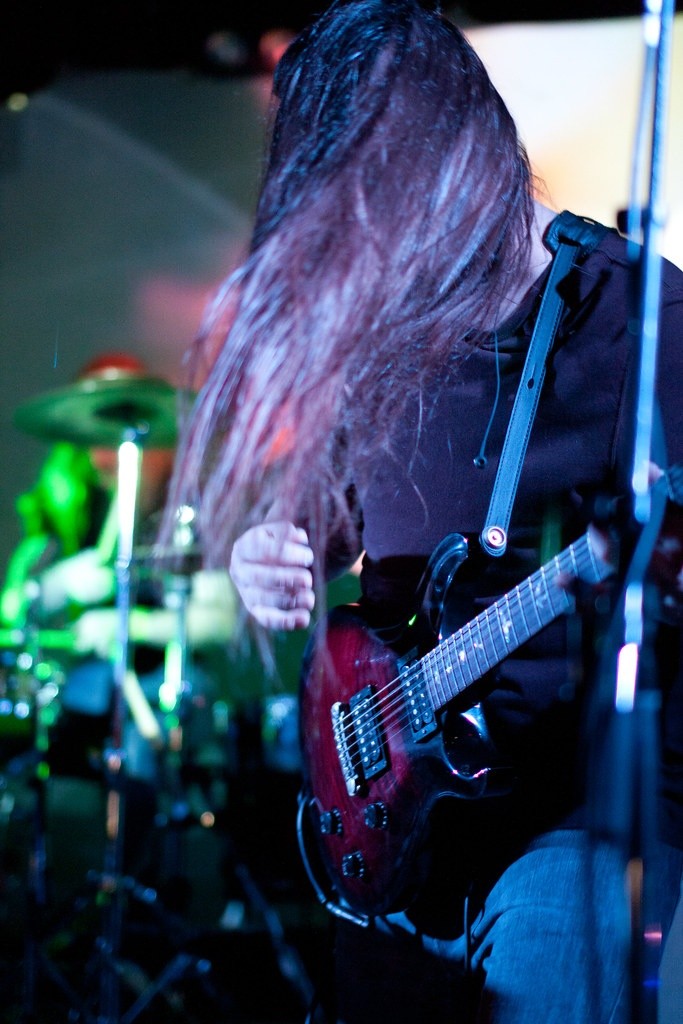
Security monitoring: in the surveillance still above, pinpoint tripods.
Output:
[36,403,222,1024]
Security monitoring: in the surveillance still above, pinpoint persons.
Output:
[147,0,682,1023]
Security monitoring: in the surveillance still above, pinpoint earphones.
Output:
[474,456,487,468]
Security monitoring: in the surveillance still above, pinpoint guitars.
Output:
[290,458,683,915]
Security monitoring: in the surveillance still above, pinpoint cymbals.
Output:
[15,378,199,443]
